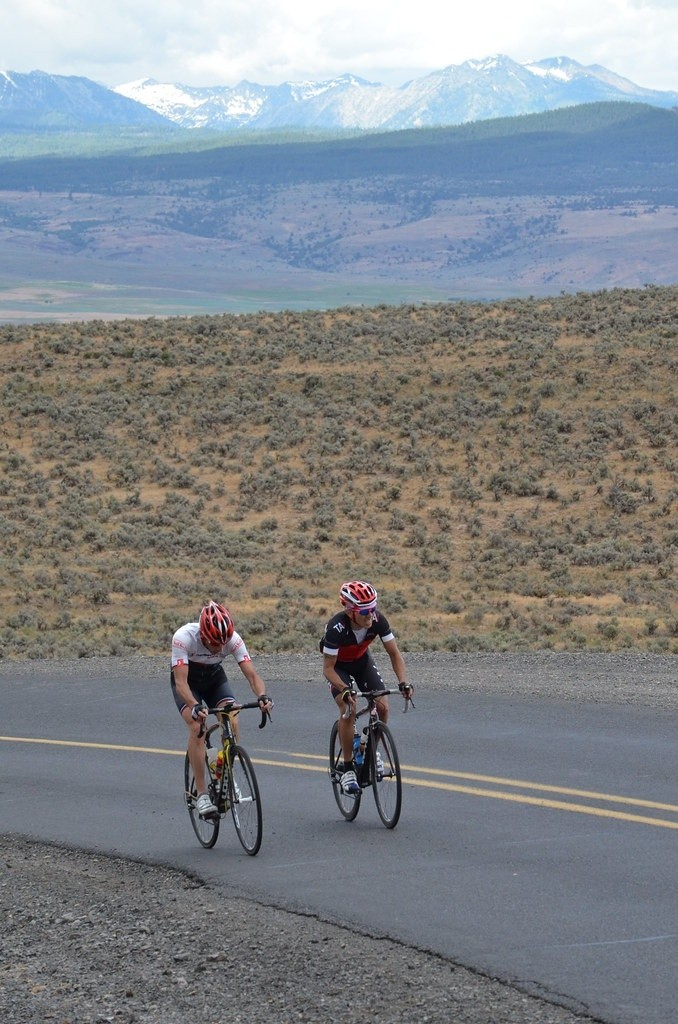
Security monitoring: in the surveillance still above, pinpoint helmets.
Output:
[339,581,378,612]
[199,599,234,646]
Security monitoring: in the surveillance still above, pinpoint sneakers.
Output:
[196,795,218,815]
[228,780,242,801]
[340,771,358,792]
[375,751,384,775]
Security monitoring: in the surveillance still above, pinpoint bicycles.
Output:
[327,679,415,829]
[182,700,273,855]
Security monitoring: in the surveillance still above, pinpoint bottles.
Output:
[206,747,218,780]
[353,733,363,764]
[216,750,224,778]
[360,733,368,755]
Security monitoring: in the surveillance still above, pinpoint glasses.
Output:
[354,607,377,616]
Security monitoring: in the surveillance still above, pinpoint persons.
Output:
[320,581,414,793]
[170,600,272,816]
